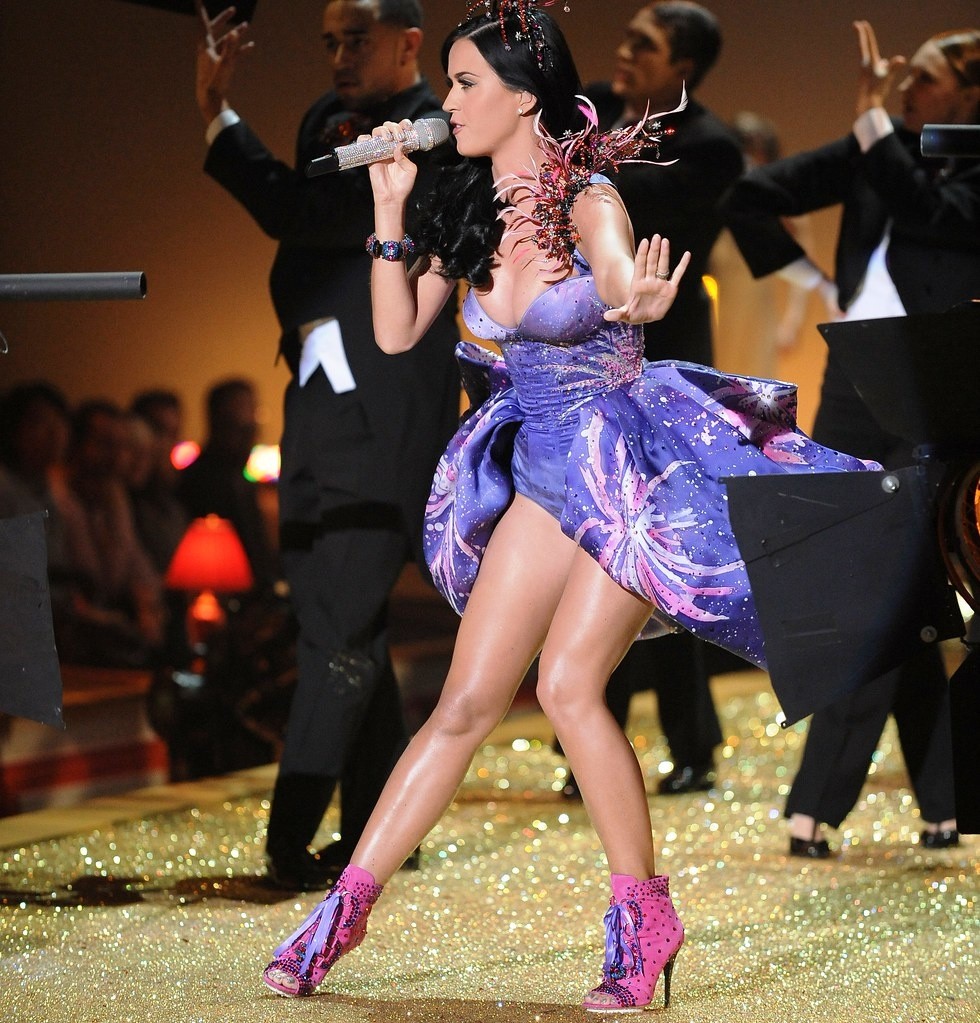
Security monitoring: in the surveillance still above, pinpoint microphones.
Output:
[305,118,448,176]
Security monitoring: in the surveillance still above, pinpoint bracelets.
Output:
[365,231,416,262]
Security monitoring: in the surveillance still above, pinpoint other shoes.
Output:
[656,755,717,792]
[317,828,421,872]
[920,826,958,849]
[265,847,329,891]
[789,836,829,856]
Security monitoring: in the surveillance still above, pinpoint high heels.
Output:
[580,871,684,1012]
[262,863,384,998]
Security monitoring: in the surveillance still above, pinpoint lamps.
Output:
[165,513,252,628]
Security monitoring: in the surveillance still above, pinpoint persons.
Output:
[195,0,466,894]
[0,374,288,790]
[706,104,819,388]
[718,15,979,868]
[547,1,853,814]
[257,1,892,1017]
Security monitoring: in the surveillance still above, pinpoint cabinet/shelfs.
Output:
[0,666,173,816]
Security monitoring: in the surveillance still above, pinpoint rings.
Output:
[655,269,670,279]
